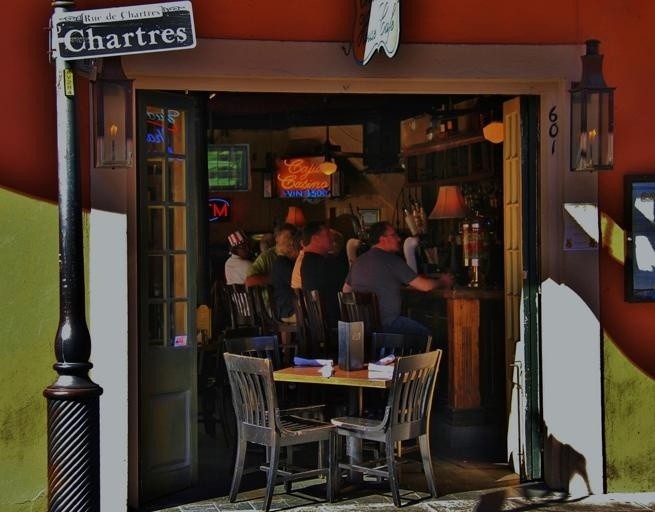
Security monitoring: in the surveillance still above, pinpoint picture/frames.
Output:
[623,174,655,303]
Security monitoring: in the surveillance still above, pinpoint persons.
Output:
[224,221,455,351]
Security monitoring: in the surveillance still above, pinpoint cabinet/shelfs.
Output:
[404,135,501,188]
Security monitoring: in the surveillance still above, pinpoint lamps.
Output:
[568,37,616,172]
[427,184,473,271]
[91,56,136,169]
[318,123,337,176]
[284,207,307,232]
[482,120,504,145]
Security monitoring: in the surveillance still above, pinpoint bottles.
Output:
[425,103,456,143]
[401,200,430,237]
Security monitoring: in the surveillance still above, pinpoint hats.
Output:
[228,230,248,247]
